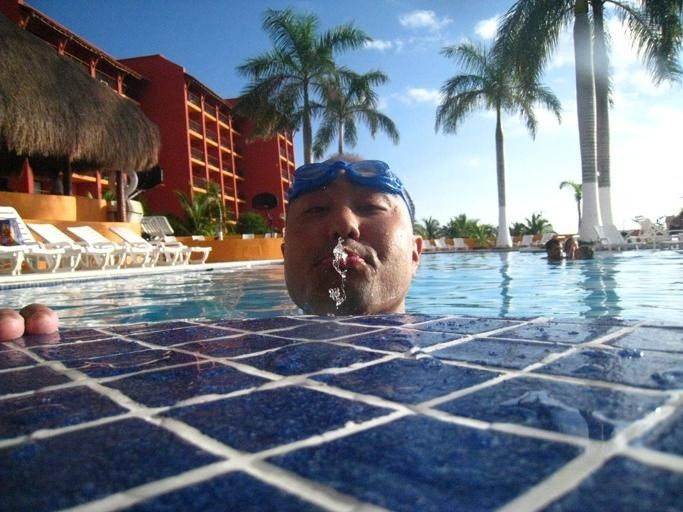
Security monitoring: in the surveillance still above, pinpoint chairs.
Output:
[421,235,469,251]
[0,205,213,267]
[592,216,683,252]
[516,232,556,250]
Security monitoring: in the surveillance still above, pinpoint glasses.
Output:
[287,160,415,221]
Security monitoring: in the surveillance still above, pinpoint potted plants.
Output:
[175,183,265,241]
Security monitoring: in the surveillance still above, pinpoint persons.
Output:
[541,238,594,259]
[0,154,424,340]
